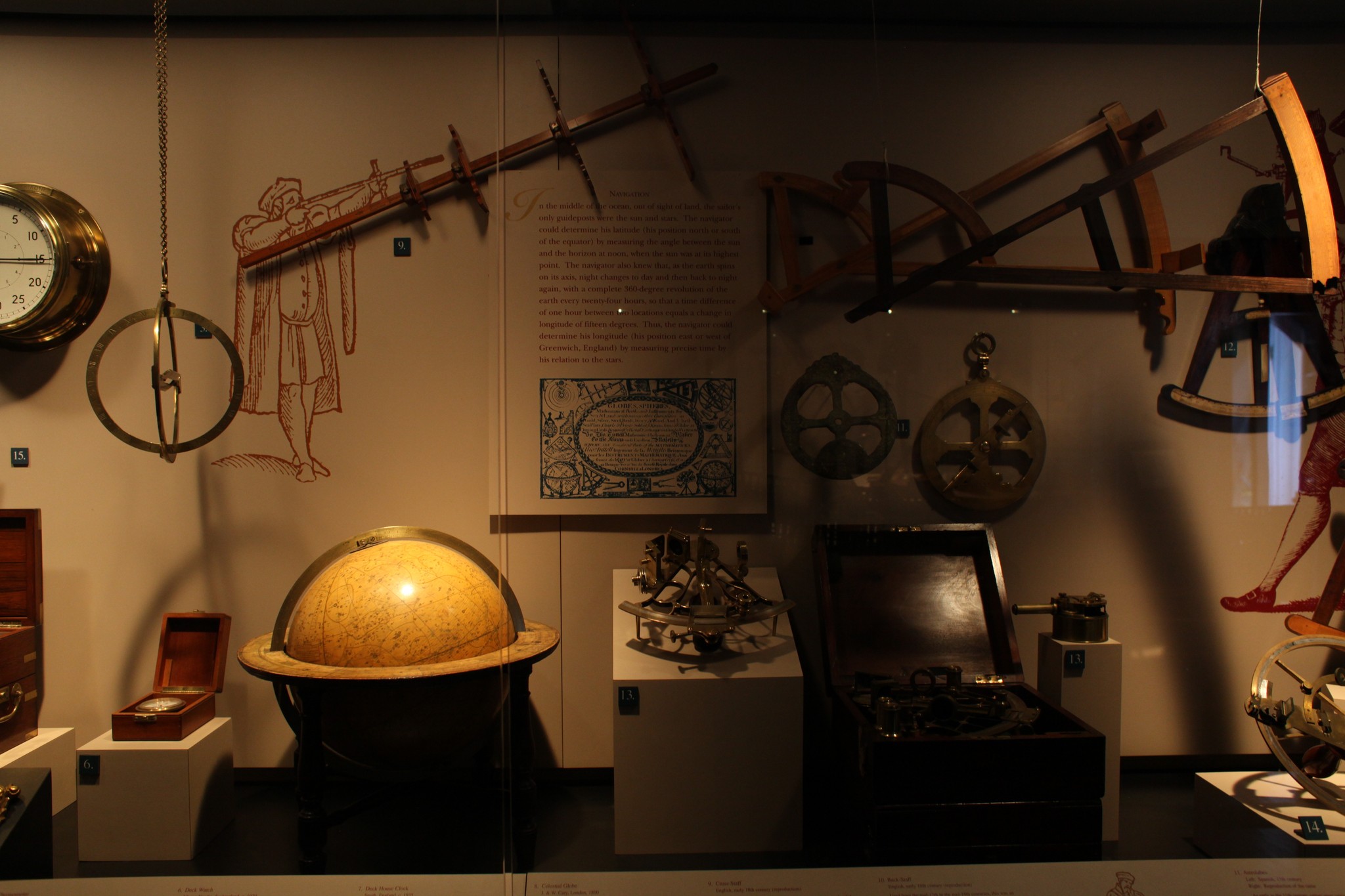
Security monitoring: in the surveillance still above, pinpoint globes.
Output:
[236,529,561,774]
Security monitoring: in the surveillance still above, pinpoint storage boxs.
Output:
[110,608,232,738]
[811,520,1107,799]
[0,507,43,754]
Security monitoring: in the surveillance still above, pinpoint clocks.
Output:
[0,183,111,348]
